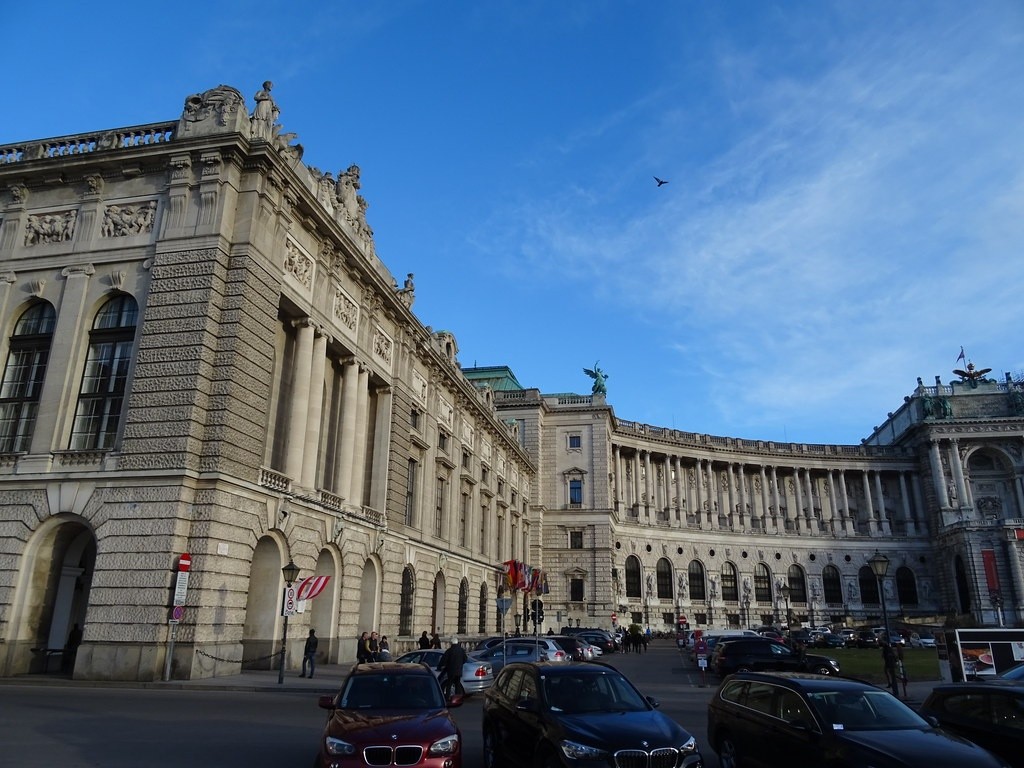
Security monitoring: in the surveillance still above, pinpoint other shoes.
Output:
[299,673,305,677]
[308,675,313,679]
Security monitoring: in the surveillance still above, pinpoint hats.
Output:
[451,638,458,644]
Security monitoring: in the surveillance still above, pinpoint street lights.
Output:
[866,549,899,699]
[745,599,750,629]
[781,581,793,647]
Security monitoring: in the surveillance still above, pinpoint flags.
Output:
[956,349,965,363]
[502,558,549,596]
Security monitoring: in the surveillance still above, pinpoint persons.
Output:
[1012,390,1024,411]
[87,176,98,192]
[66,622,83,666]
[505,632,509,637]
[8,184,23,201]
[651,630,678,640]
[419,631,441,649]
[357,631,389,664]
[615,625,652,654]
[103,201,157,237]
[298,629,318,678]
[881,641,910,688]
[948,480,956,499]
[373,330,391,364]
[25,210,77,246]
[921,394,954,417]
[547,627,554,635]
[335,290,356,330]
[250,81,276,146]
[438,638,467,702]
[959,442,1020,460]
[284,241,311,287]
[583,360,606,393]
[405,273,415,309]
[617,572,934,602]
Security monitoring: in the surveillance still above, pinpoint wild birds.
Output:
[653,176,669,187]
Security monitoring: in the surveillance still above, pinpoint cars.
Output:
[684,627,785,664]
[394,648,492,699]
[794,626,937,649]
[918,661,1024,768]
[470,630,622,673]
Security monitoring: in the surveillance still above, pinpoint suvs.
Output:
[481,661,704,768]
[320,661,464,768]
[707,669,1001,768]
[710,637,839,683]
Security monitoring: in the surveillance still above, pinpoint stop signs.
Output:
[679,616,687,624]
[612,614,616,620]
[179,554,192,573]
